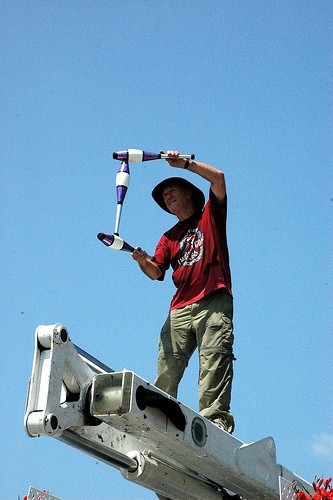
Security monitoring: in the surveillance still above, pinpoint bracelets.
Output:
[183,159,190,170]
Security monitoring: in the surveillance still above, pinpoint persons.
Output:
[130,149,238,436]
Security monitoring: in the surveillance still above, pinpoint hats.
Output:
[151,177,205,217]
